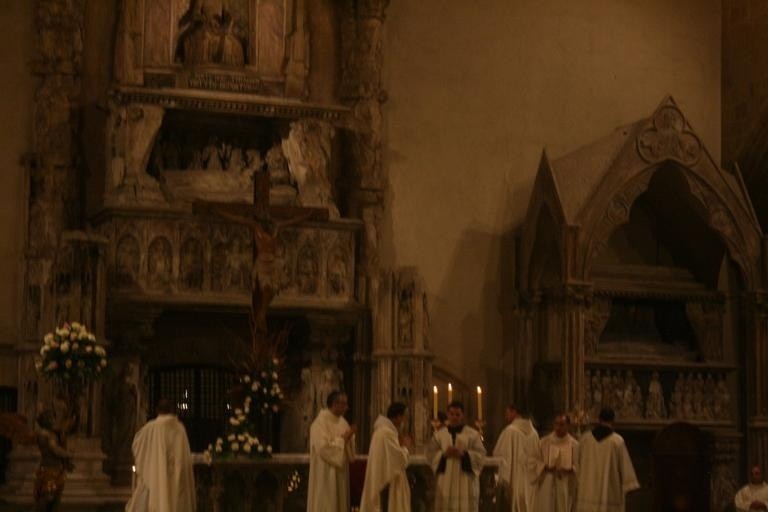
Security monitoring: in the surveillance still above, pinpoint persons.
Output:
[539,411,582,512]
[35,409,71,512]
[734,464,768,512]
[124,399,197,511]
[591,368,716,420]
[422,400,488,512]
[490,402,539,512]
[574,406,641,512]
[360,401,414,512]
[304,390,357,512]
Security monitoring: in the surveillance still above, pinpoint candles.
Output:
[432,384,440,422]
[476,385,483,422]
[448,381,453,407]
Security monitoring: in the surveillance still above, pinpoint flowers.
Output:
[34,321,114,435]
[203,352,285,466]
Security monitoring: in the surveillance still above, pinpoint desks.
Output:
[190,451,501,512]
